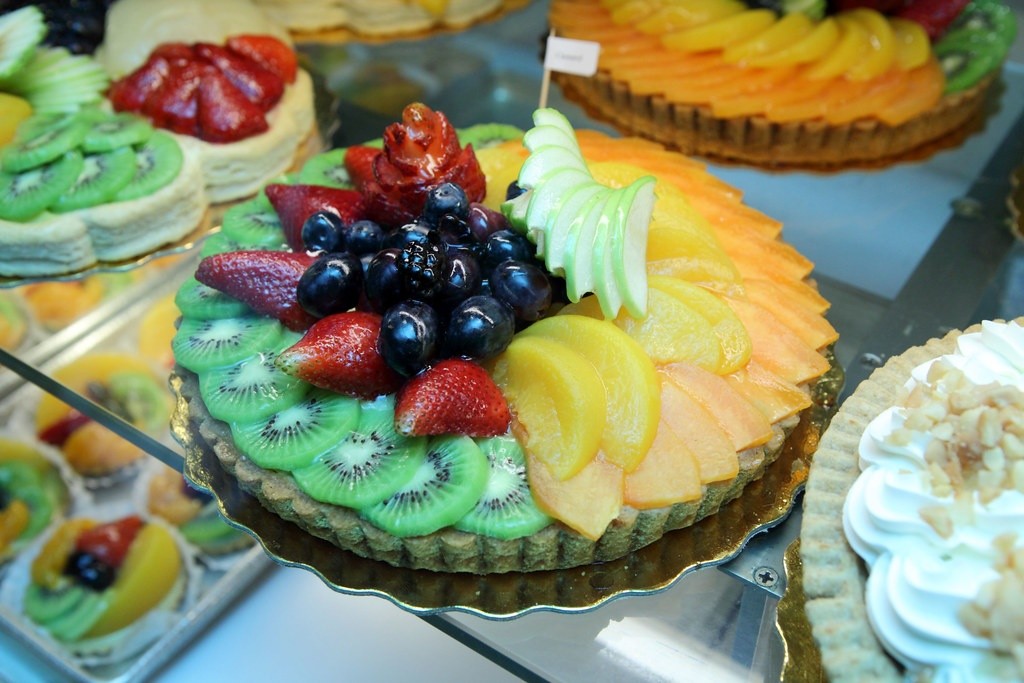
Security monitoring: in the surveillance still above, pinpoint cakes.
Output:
[0,0,537,286]
[797,317,1024,683]
[0,238,263,664]
[167,103,847,617]
[547,0,1017,167]
